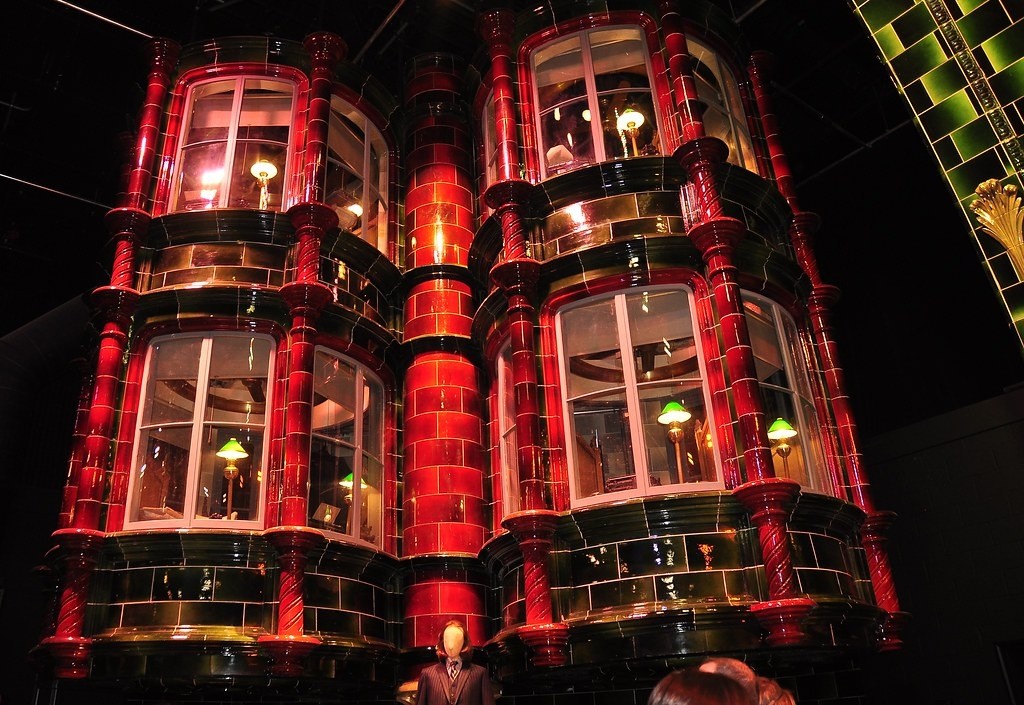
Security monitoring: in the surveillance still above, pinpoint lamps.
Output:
[617,108,645,157]
[339,471,367,535]
[348,204,363,235]
[250,158,278,210]
[657,400,692,483]
[216,437,249,520]
[767,417,798,478]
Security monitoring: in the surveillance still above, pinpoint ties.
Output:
[450,661,459,695]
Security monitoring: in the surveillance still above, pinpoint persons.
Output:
[650,657,796,705]
[417,621,493,705]
